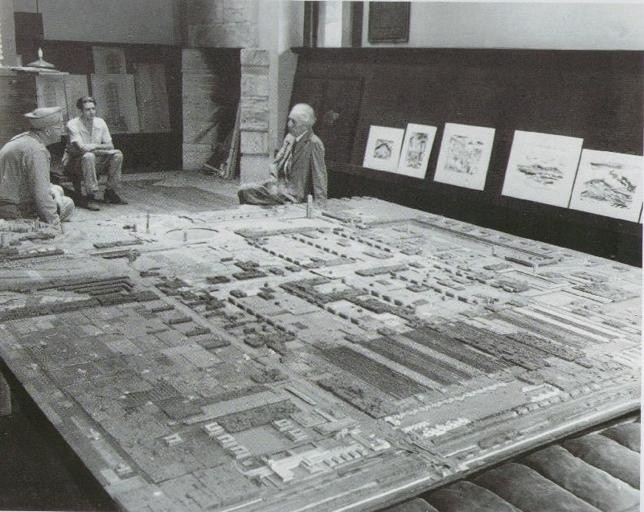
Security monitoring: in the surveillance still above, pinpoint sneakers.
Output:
[80,188,128,210]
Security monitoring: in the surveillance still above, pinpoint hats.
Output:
[24,106,64,128]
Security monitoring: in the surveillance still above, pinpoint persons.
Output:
[0,106,76,226]
[238,103,327,215]
[61,97,128,210]
